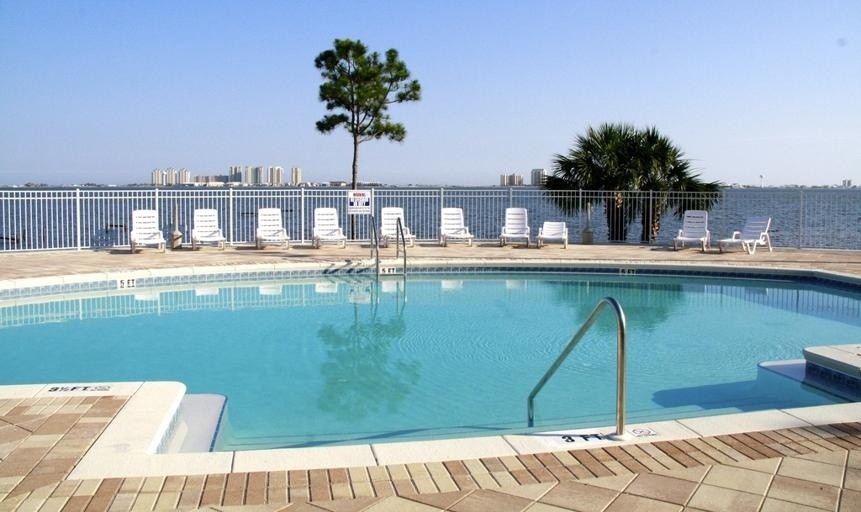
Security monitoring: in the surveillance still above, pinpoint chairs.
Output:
[381,206,417,248]
[715,216,775,255]
[536,222,569,249]
[131,210,167,253]
[673,210,712,252]
[192,209,227,250]
[499,208,531,248]
[312,208,347,249]
[256,208,291,249]
[440,208,475,247]
